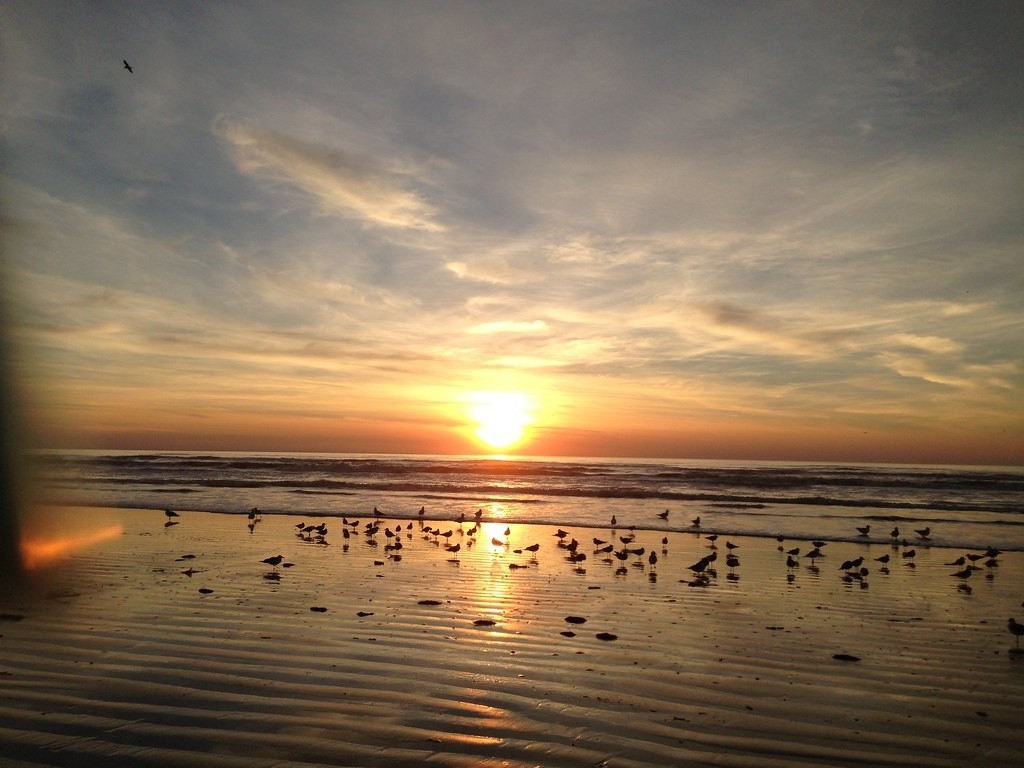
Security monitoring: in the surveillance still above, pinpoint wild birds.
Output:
[158,506,1023,642]
